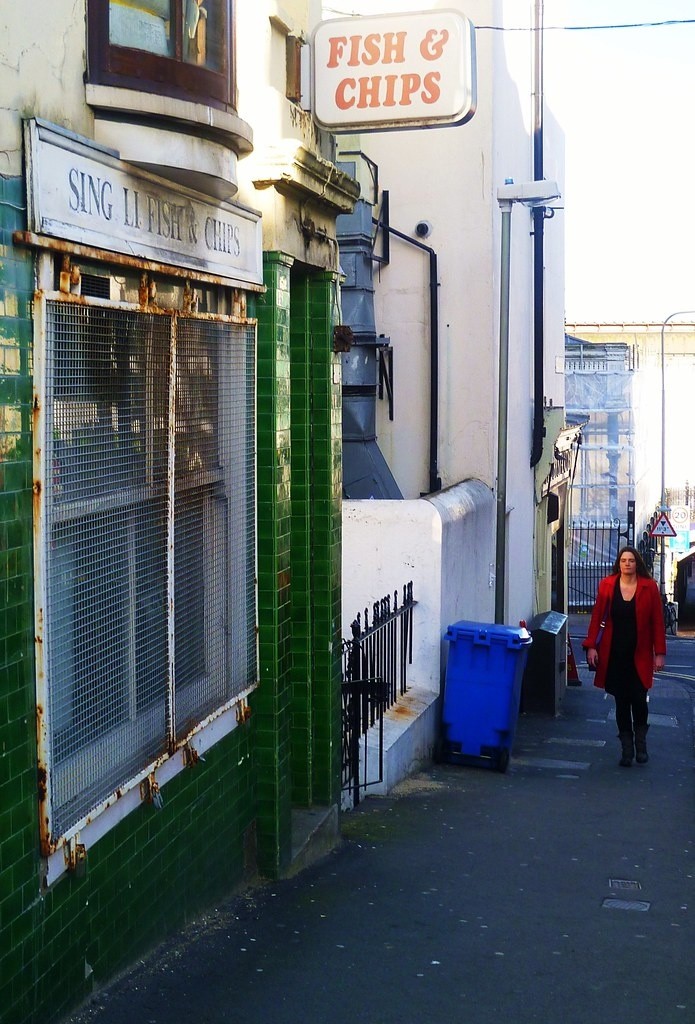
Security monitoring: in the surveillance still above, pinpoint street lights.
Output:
[491,177,566,625]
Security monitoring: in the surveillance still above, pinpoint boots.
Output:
[635,731,648,763]
[619,738,635,766]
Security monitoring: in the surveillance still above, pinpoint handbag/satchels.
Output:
[586,650,596,671]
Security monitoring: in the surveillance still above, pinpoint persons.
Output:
[583,548,666,767]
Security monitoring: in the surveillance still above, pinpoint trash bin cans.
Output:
[436,619,534,774]
